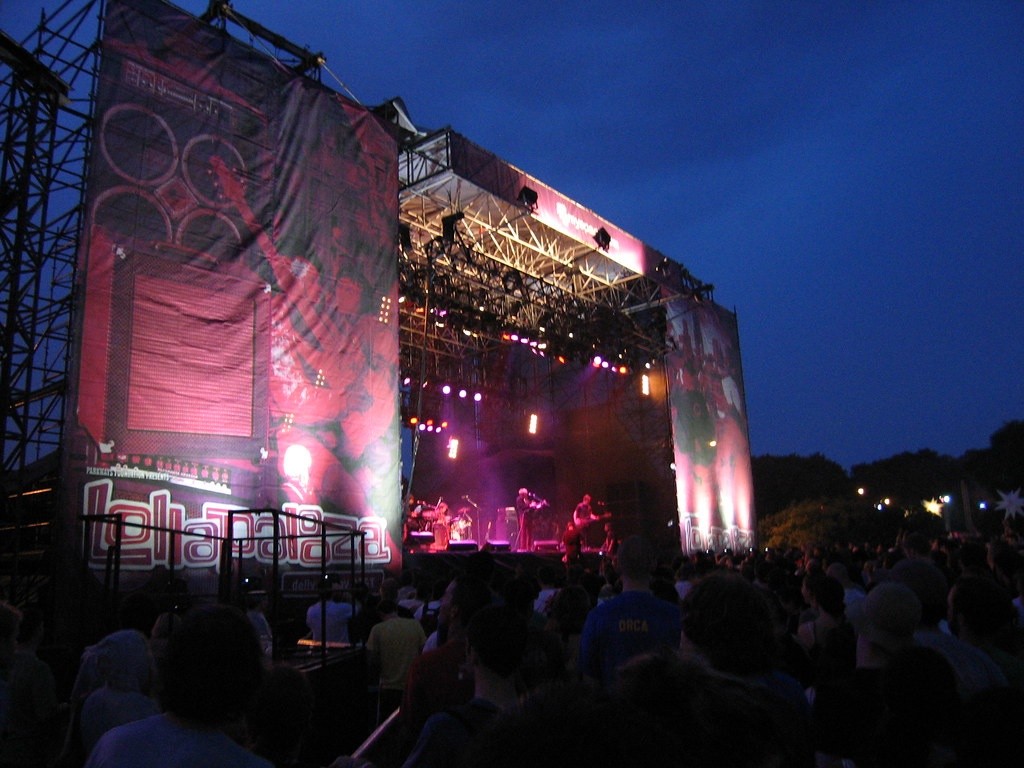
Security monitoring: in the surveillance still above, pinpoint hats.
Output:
[82,630,149,684]
[151,612,183,638]
[845,581,919,651]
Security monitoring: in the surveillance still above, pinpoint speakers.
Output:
[479,539,511,552]
[447,540,478,551]
[402,531,435,551]
[531,539,559,553]
[496,519,519,549]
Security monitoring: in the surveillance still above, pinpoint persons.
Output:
[0,529,1024,768]
[433,505,462,546]
[402,495,427,544]
[517,488,545,551]
[574,496,598,549]
